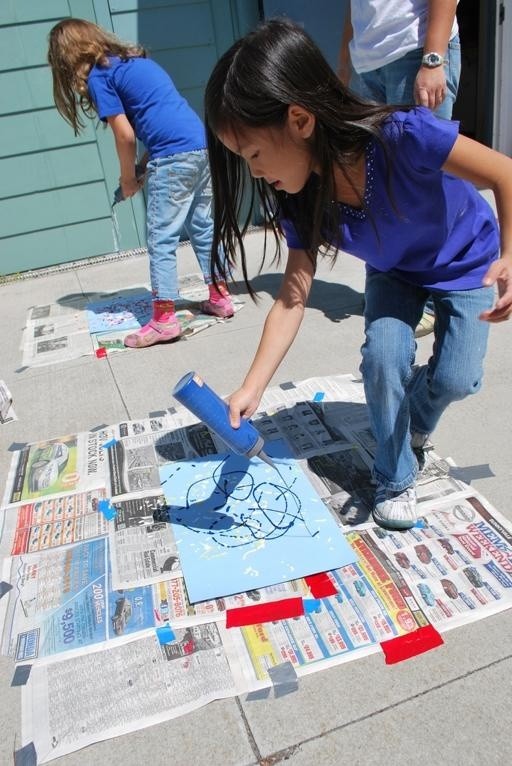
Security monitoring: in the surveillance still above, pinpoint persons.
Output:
[208,15,511,528]
[338,0,462,121]
[51,19,235,348]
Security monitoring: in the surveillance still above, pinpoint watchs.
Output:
[421,52,445,68]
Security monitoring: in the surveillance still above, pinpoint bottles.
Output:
[111,165,145,208]
[172,371,279,472]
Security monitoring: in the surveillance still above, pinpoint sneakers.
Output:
[406,368,433,452]
[124,313,182,348]
[197,296,234,318]
[372,468,419,529]
[406,303,439,339]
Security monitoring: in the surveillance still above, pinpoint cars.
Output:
[31,442,70,494]
[373,515,450,549]
[112,597,133,636]
[415,544,432,564]
[215,580,367,632]
[441,578,459,600]
[440,539,453,555]
[465,567,485,589]
[417,582,435,607]
[395,552,411,570]
